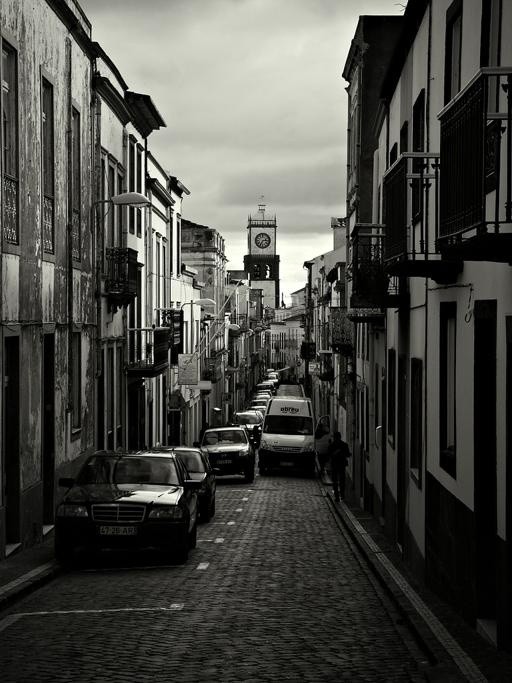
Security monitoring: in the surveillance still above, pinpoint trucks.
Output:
[255,397,331,481]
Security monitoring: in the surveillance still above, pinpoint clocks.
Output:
[255,232,271,248]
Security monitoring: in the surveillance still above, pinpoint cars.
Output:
[50,447,200,564]
[146,441,216,522]
[196,363,280,484]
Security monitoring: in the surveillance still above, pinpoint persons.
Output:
[327,431,350,502]
